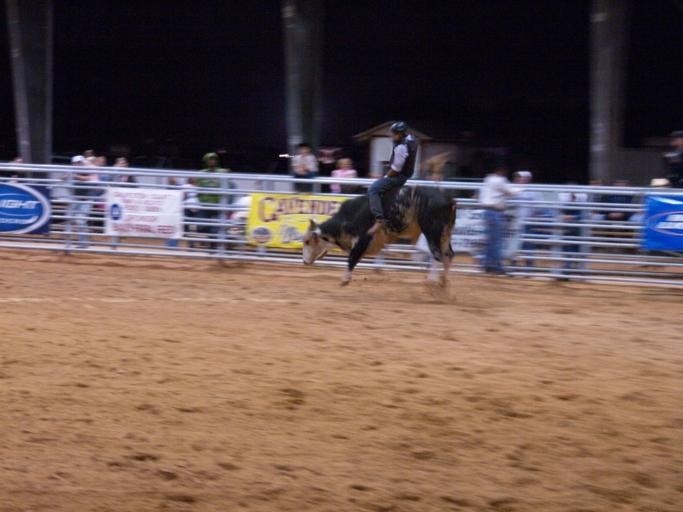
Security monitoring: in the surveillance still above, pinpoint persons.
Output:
[161,151,234,253]
[658,130,683,188]
[8,157,27,182]
[366,120,418,235]
[289,143,357,191]
[61,149,139,252]
[479,165,649,281]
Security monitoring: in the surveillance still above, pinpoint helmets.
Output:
[389,121,408,135]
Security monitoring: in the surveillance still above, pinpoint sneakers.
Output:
[366,221,385,235]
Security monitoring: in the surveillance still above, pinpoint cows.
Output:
[300,184,510,290]
